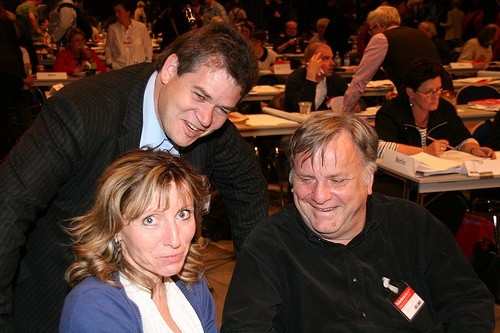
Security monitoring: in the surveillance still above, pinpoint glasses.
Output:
[71,39,86,43]
[416,88,445,97]
[367,26,378,35]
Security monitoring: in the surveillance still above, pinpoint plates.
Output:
[228,110,249,126]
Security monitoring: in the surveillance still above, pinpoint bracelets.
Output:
[470,146,477,154]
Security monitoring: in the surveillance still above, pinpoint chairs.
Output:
[457,85,500,105]
[472,119,500,151]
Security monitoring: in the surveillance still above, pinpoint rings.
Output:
[440,146,443,150]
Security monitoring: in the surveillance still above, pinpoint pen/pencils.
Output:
[426,136,455,150]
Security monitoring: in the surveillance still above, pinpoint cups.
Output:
[299,101,312,116]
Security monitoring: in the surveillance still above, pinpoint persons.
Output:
[55,148,220,333]
[0,0,500,163]
[222,107,496,332]
[0,20,270,332]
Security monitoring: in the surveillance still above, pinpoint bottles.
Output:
[334,52,340,66]
[344,56,349,66]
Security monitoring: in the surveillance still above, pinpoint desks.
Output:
[227,54,500,206]
[32,75,81,85]
